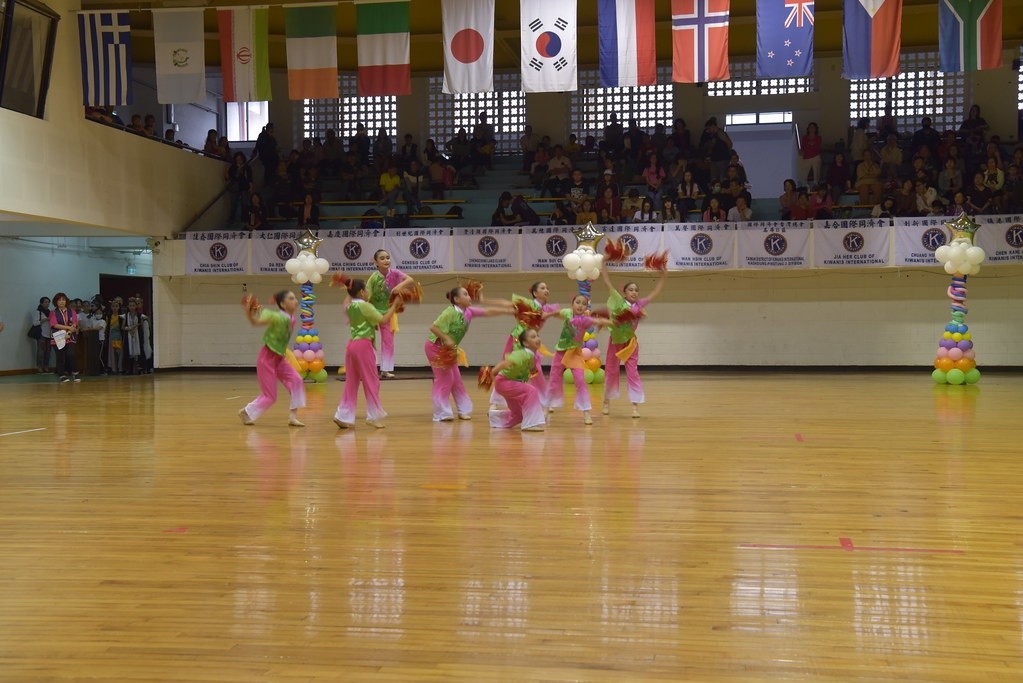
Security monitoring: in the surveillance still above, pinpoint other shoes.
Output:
[584,415,592,425]
[73,374,81,382]
[238,409,254,425]
[549,408,554,413]
[602,401,609,414]
[288,419,305,427]
[366,418,385,428]
[381,371,395,378]
[522,426,544,431]
[459,414,471,420]
[631,410,640,418]
[334,417,349,428]
[61,376,70,383]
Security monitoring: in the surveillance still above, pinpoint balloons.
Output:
[928,212,986,385]
[561,249,604,281]
[562,327,605,385]
[284,229,328,382]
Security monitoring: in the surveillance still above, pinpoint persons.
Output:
[539,294,614,425]
[31,292,153,383]
[488,329,546,432]
[94,105,1023,231]
[598,263,665,418]
[487,281,569,423]
[364,249,413,378]
[424,287,516,421]
[239,290,307,427]
[334,279,403,430]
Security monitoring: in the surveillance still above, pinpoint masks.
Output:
[96,315,103,319]
[713,187,721,192]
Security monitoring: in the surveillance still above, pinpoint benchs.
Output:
[832,140,1023,219]
[251,153,707,225]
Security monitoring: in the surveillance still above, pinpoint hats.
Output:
[603,169,612,175]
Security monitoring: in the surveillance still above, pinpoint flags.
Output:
[76,9,133,106]
[938,0,1004,72]
[281,0,814,99]
[215,5,273,102]
[151,8,207,105]
[841,0,903,79]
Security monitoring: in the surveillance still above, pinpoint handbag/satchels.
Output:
[28,325,42,340]
